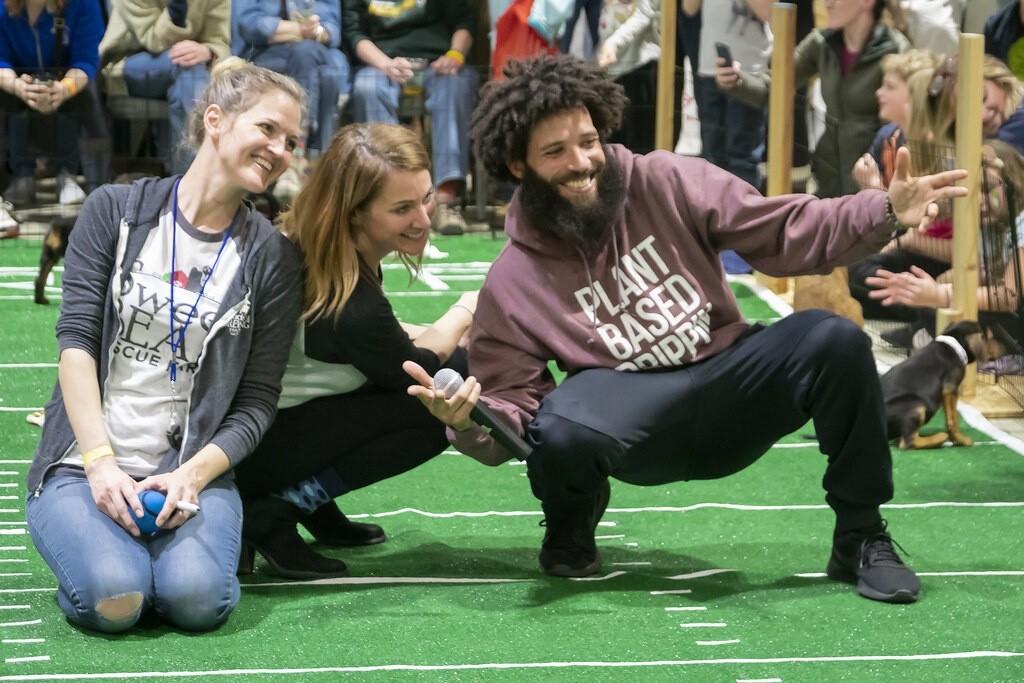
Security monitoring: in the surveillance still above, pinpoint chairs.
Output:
[105,94,169,178]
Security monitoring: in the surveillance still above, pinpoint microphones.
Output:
[434,368,533,463]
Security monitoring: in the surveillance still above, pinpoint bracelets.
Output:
[62,78,76,95]
[449,305,473,315]
[886,199,907,231]
[317,26,324,41]
[81,446,114,464]
[446,50,465,64]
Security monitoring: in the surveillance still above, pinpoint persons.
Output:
[849,0,1024,373]
[343,0,484,233]
[99,0,233,171]
[231,0,349,156]
[237,122,482,577]
[496,0,684,155]
[672,0,913,198]
[27,54,308,634]
[0,0,111,234]
[403,54,968,599]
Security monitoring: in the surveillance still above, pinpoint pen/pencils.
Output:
[177,501,200,514]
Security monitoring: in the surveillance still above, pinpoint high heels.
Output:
[291,499,386,546]
[236,524,346,579]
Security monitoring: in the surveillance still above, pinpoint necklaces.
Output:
[168,177,241,450]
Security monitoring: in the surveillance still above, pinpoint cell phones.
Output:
[715,42,732,68]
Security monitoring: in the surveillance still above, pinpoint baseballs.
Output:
[128,490,169,533]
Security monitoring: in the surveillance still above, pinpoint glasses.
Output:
[926,57,959,99]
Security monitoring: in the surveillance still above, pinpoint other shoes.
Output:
[272,166,302,202]
[878,319,935,349]
[430,202,470,234]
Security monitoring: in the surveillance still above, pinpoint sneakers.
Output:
[2,176,36,206]
[538,519,600,576]
[825,519,922,604]
[54,169,87,205]
[0,196,21,239]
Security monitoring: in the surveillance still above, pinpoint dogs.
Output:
[35,216,78,305]
[878,321,1007,452]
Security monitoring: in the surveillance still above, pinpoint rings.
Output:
[926,201,940,216]
